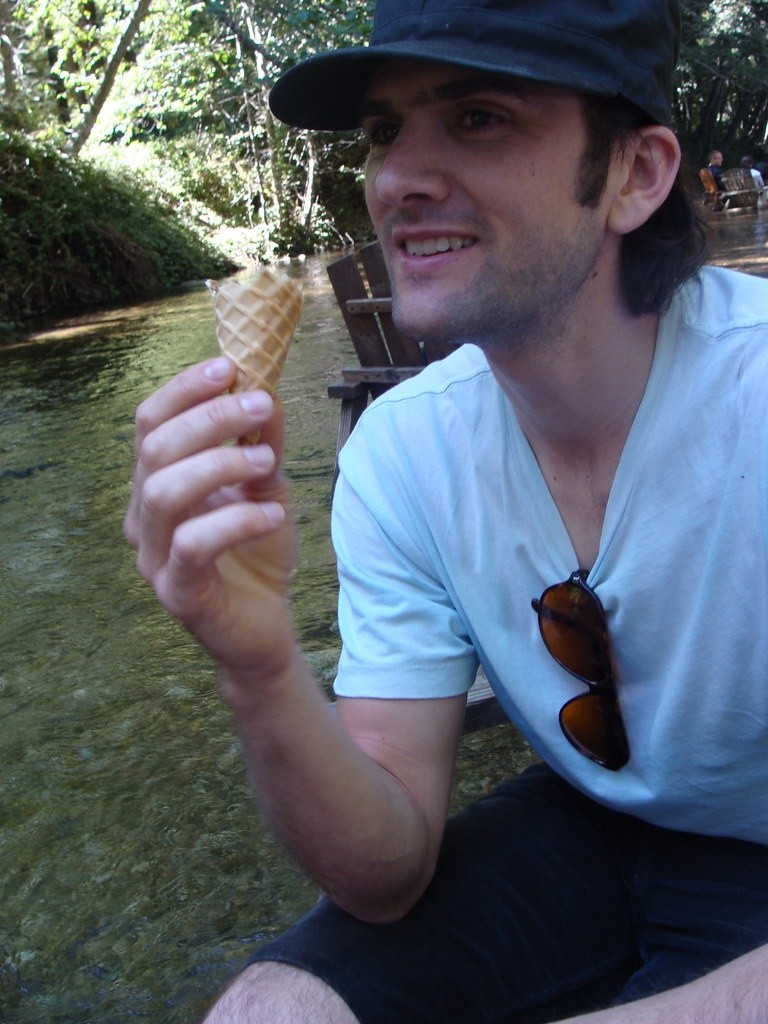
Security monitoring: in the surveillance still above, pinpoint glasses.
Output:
[532,570,630,771]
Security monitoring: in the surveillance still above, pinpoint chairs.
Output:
[719,168,768,215]
[326,238,459,510]
[700,168,725,212]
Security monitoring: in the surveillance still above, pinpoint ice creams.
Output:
[210,264,302,452]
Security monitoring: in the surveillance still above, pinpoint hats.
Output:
[269,0,680,128]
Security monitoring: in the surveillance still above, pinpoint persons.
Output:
[738,156,763,190]
[125,0,768,1024]
[707,151,726,191]
